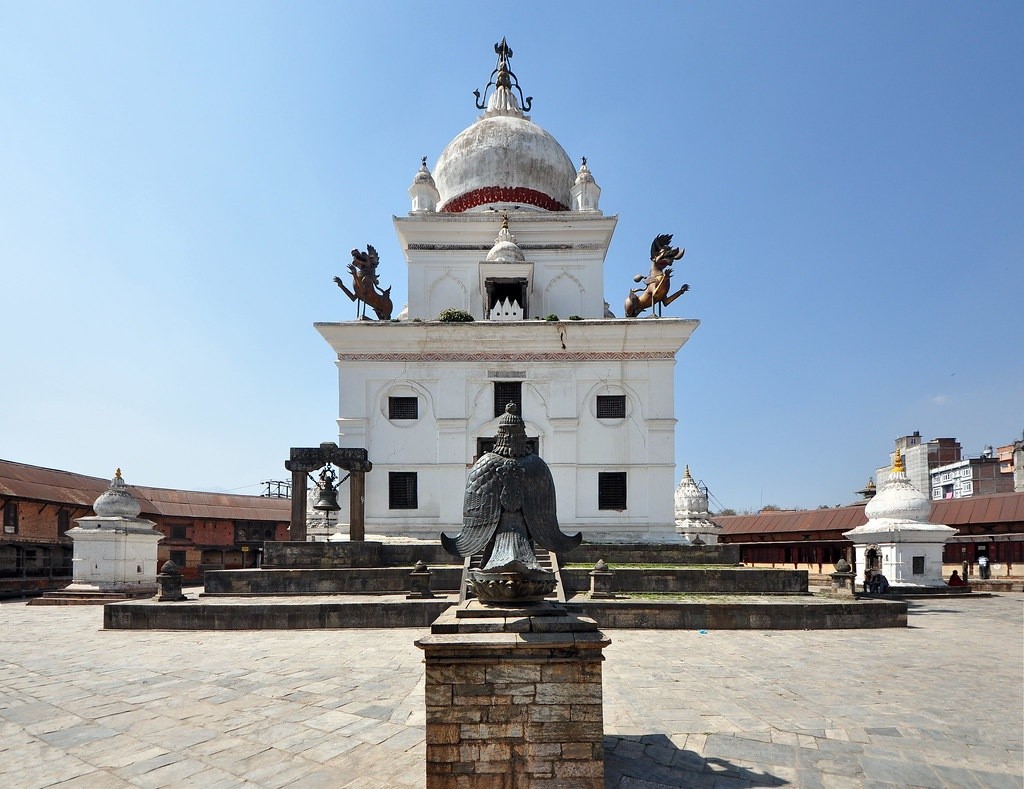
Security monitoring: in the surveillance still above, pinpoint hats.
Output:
[864,569,870,575]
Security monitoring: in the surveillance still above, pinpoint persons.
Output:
[863,572,890,594]
[948,570,964,586]
